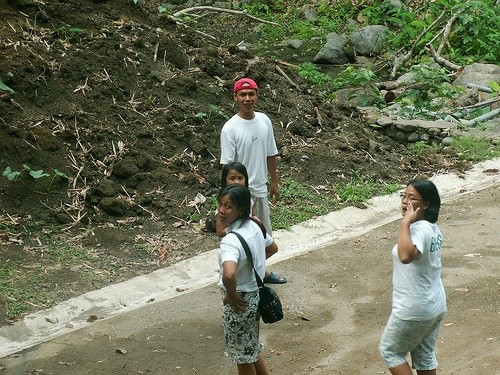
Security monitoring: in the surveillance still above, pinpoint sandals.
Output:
[263,272,287,283]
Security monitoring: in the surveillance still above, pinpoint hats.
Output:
[234,78,258,94]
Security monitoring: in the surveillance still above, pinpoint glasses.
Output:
[400,192,424,202]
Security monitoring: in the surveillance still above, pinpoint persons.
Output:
[220,78,287,284]
[216,162,278,375]
[379,179,447,375]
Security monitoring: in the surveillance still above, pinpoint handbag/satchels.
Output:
[258,287,283,324]
